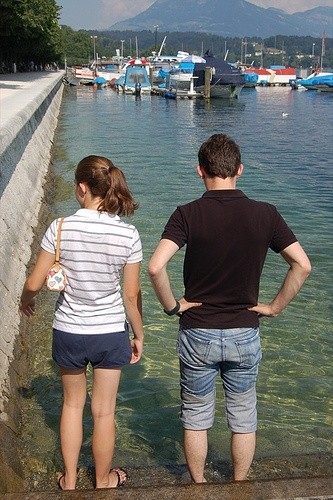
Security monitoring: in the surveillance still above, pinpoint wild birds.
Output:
[282,112,289,116]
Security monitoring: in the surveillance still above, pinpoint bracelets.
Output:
[162,301,181,315]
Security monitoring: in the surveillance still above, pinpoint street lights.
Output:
[90,35,98,59]
[153,24,159,51]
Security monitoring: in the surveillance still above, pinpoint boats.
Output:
[289,71,333,91]
[114,64,151,95]
[93,77,108,90]
[69,52,296,88]
[298,69,333,93]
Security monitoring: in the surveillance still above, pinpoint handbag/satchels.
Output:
[46,217,66,292]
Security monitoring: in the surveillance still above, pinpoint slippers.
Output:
[57,470,67,490]
[109,466,128,488]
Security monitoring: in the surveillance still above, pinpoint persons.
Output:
[148,134,312,484]
[24,154,147,492]
